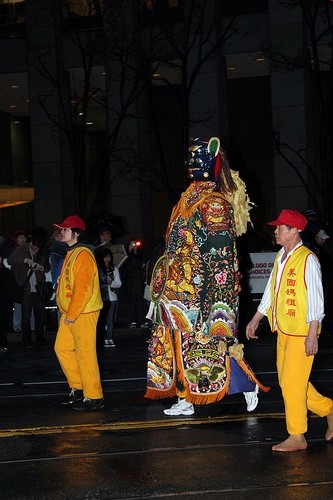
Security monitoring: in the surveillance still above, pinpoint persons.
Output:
[53,215,104,410]
[312,226,333,286]
[0,225,165,352]
[246,209,333,451]
[144,137,271,415]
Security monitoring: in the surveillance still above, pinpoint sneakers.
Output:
[104,339,116,347]
[58,388,84,405]
[72,398,105,412]
[243,384,259,411]
[163,398,195,415]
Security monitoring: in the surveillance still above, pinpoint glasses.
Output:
[59,228,70,231]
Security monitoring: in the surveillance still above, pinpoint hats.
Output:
[53,215,86,230]
[316,230,330,240]
[267,209,308,231]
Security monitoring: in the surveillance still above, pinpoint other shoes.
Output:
[24,345,32,352]
[0,347,8,352]
[140,322,149,328]
[130,322,137,328]
[325,428,333,441]
[272,437,308,451]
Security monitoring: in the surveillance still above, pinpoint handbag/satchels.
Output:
[143,284,152,301]
[11,282,26,305]
